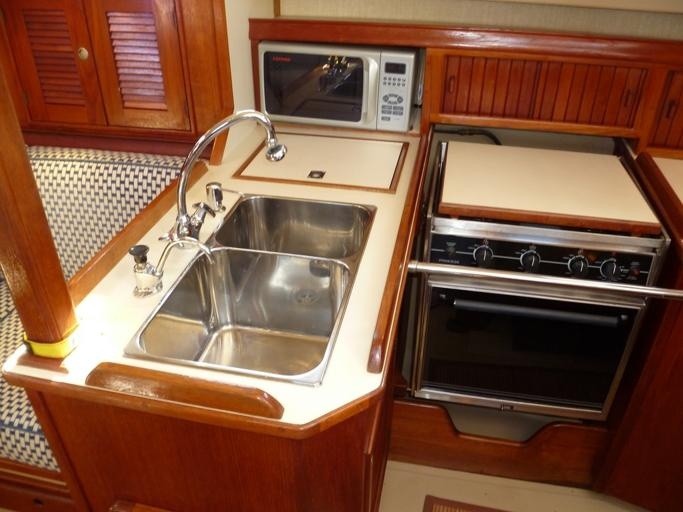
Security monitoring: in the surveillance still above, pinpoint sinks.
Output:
[124,247,350,385]
[210,192,378,259]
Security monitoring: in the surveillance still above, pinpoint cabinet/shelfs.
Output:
[0,0,192,134]
[433,49,647,132]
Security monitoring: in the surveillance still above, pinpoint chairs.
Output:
[1,144,193,496]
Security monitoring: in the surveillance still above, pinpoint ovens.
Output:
[407,262,667,424]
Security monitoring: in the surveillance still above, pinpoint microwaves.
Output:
[254,39,417,133]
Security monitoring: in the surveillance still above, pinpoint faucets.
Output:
[176,109,287,217]
[156,236,214,276]
